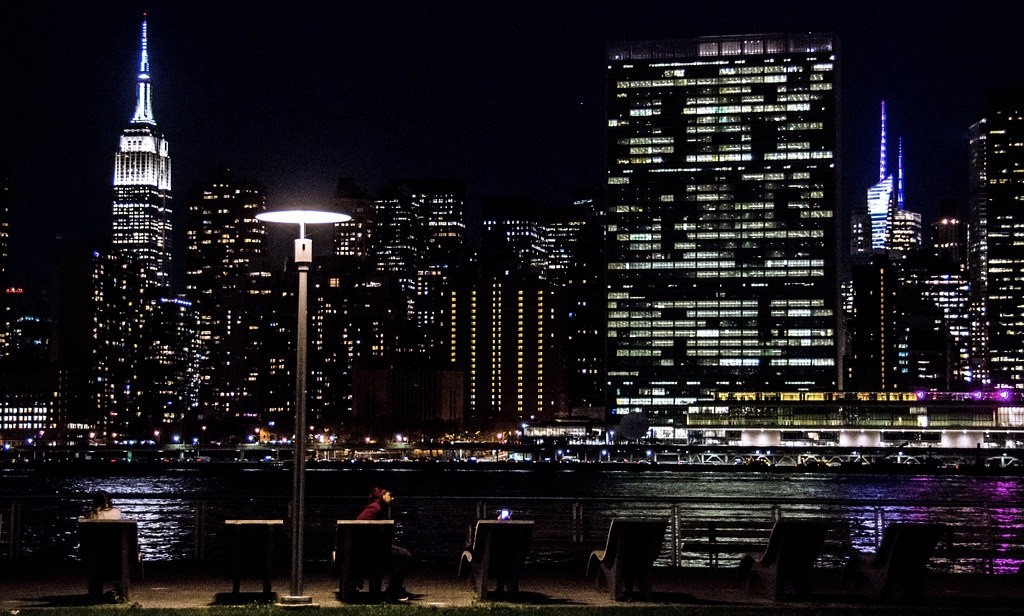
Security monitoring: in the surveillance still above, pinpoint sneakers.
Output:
[385,587,414,602]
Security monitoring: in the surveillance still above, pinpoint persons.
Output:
[356,486,412,600]
[495,507,513,595]
[84,490,124,523]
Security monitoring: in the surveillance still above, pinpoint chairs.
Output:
[221,519,287,597]
[584,519,668,600]
[78,519,140,600]
[330,520,397,605]
[458,518,535,603]
[859,523,948,597]
[741,518,832,602]
